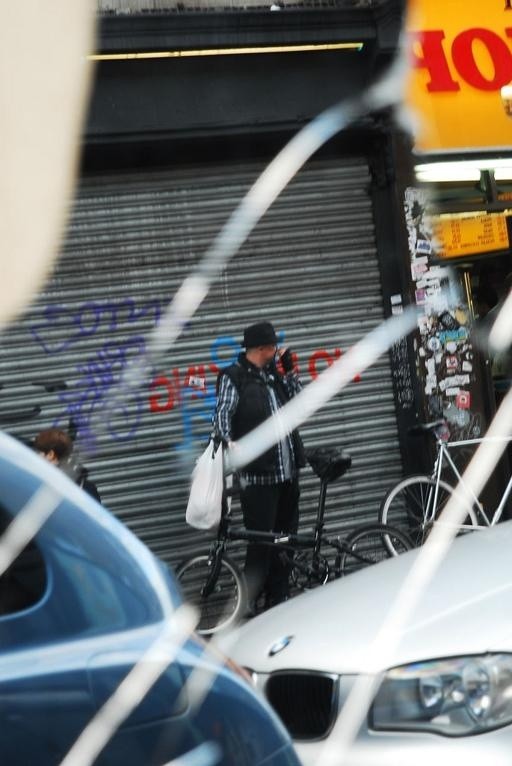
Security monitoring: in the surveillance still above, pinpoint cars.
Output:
[214,519,508,758]
[0,422,306,766]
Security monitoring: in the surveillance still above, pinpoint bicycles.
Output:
[378,417,512,556]
[173,433,416,638]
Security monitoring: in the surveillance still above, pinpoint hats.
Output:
[239,321,283,347]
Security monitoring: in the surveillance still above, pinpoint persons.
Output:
[30,430,101,507]
[209,320,305,628]
